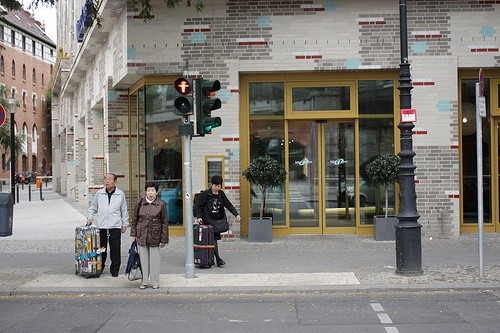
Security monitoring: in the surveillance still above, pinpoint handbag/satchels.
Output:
[209,215,230,233]
[125,240,143,280]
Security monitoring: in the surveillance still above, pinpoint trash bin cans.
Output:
[0,192,14,237]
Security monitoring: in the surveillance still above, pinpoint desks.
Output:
[36,176,52,187]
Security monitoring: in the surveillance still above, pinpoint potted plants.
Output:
[365,154,401,241]
[243,155,288,242]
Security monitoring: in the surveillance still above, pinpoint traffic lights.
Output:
[196,78,222,136]
[173,77,194,116]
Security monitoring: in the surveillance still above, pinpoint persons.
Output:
[130,182,169,289]
[87,173,129,277]
[195,176,240,267]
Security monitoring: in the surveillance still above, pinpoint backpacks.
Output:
[193,190,210,217]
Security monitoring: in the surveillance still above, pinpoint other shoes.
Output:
[112,272,118,277]
[139,284,147,289]
[217,259,225,267]
[153,284,159,289]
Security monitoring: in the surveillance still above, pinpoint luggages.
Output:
[74,223,102,279]
[193,220,215,268]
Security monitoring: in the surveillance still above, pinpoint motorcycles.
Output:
[15,173,31,184]
